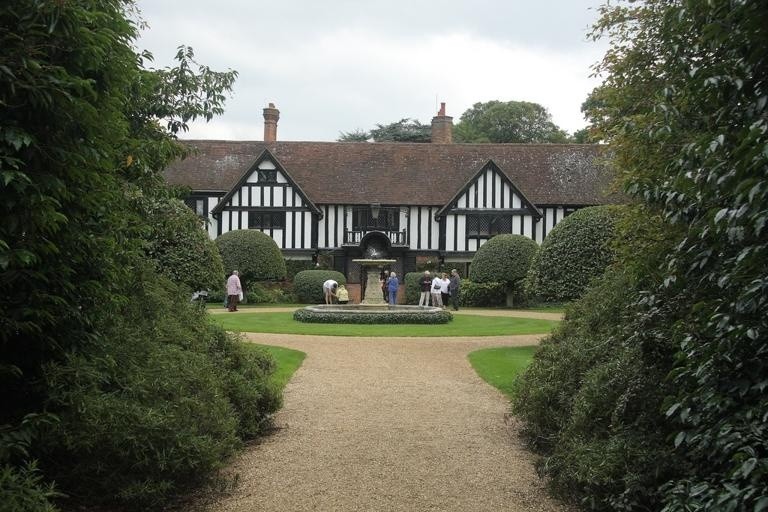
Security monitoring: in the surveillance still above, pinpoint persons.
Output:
[430,272,443,308]
[323,279,338,304]
[226,270,242,312]
[383,272,399,305]
[450,269,460,311]
[440,273,451,310]
[379,270,390,303]
[336,284,349,304]
[419,271,432,306]
